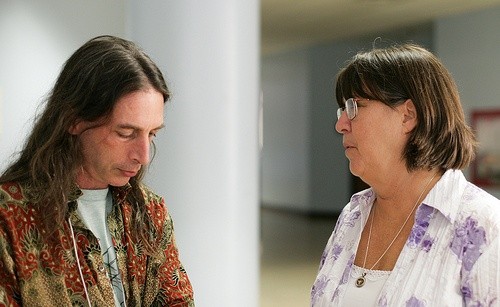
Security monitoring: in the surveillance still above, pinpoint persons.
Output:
[310,42,500,307]
[0,35,197,307]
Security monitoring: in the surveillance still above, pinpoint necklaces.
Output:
[355,169,436,287]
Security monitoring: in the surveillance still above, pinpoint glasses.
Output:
[336,97,411,122]
